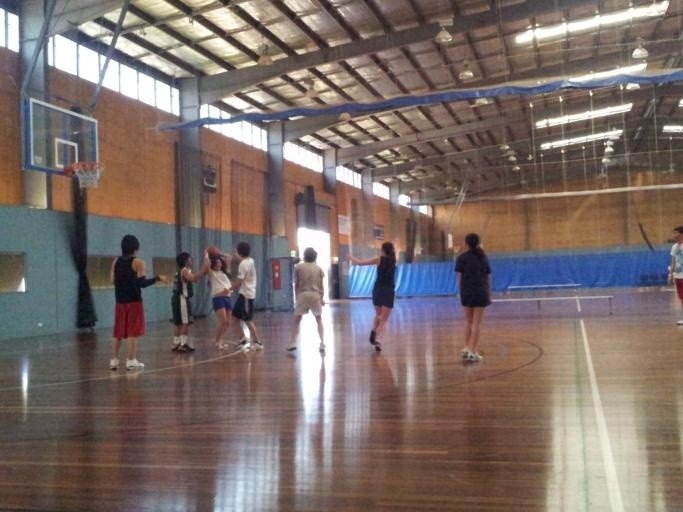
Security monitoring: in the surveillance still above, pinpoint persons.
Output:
[109,235,169,370]
[223,242,264,349]
[285,247,326,352]
[454,233,493,362]
[171,252,209,352]
[668,226,683,325]
[346,241,400,350]
[203,248,234,350]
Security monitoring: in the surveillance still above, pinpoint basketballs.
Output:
[207,246,220,256]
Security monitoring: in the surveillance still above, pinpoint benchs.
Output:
[492,294,615,316]
[507,282,584,298]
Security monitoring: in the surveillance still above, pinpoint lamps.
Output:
[177,1,680,108]
[394,86,683,194]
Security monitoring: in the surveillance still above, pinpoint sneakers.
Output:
[319,343,325,351]
[370,328,382,351]
[214,338,264,349]
[109,359,118,370]
[463,350,482,361]
[170,343,194,351]
[126,359,145,369]
[285,345,296,351]
